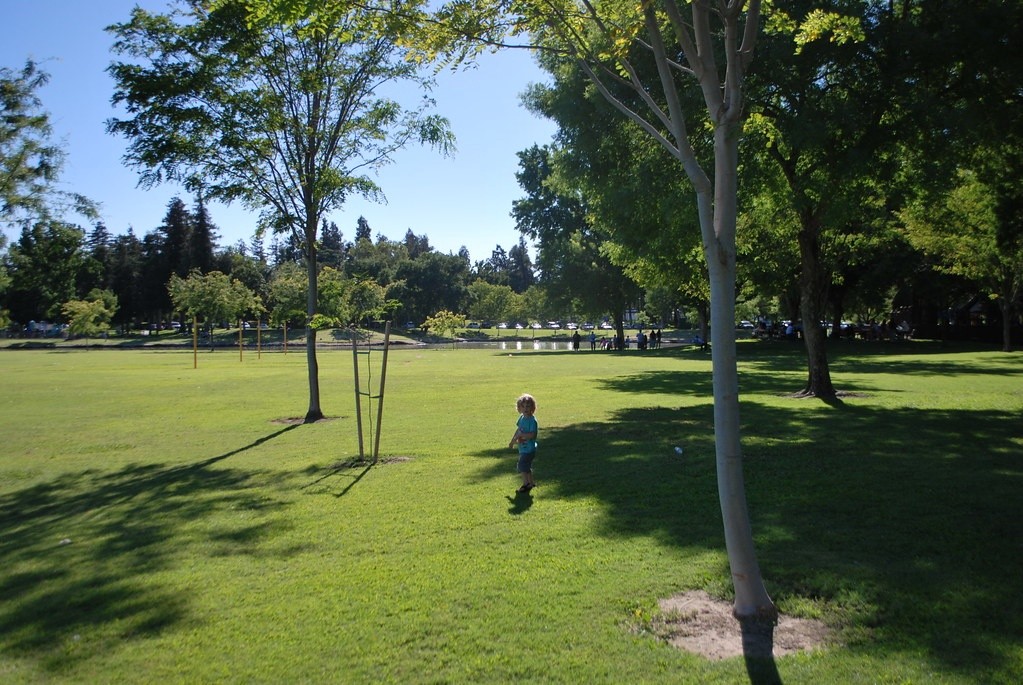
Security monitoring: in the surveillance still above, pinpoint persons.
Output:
[693,334,706,350]
[754,321,916,342]
[600,335,617,351]
[590,332,595,351]
[509,393,538,492]
[624,329,661,350]
[573,331,581,352]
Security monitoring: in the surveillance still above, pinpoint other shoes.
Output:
[518,482,535,491]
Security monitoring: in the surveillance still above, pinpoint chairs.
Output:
[906,329,915,340]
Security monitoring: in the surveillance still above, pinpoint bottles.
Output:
[675,446,682,454]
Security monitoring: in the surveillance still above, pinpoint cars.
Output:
[240,322,250,327]
[145,321,181,329]
[564,323,594,330]
[599,323,612,329]
[466,322,478,328]
[404,321,415,329]
[480,323,491,329]
[258,324,270,329]
[496,323,524,329]
[614,321,664,329]
[547,321,561,329]
[739,320,755,329]
[762,320,855,330]
[530,322,542,328]
